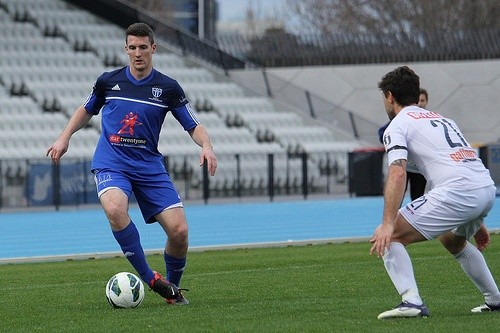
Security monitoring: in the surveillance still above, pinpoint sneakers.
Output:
[471,301,500,313]
[376,301,430,320]
[149,270,192,306]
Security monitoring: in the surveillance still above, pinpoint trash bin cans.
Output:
[348,147,383,197]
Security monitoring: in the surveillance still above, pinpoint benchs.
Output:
[0,1,370,207]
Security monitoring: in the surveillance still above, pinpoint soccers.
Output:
[106,272,144,308]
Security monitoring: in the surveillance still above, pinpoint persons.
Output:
[46,23,217,306]
[369,66,500,320]
